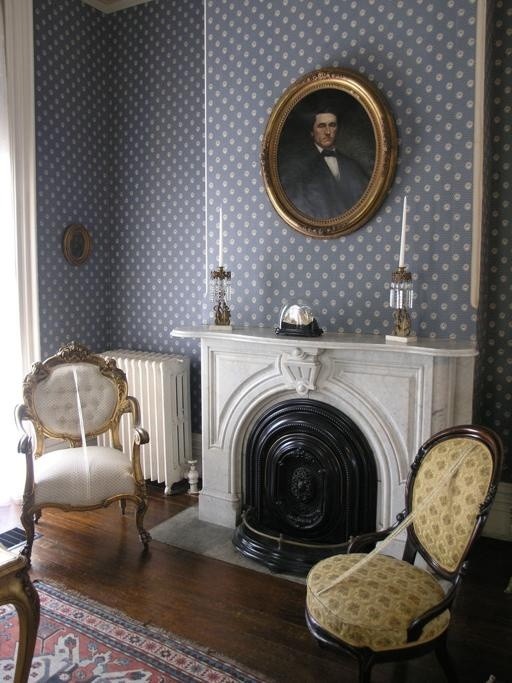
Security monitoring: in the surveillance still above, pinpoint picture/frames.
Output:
[62,223,92,266]
[259,68,399,240]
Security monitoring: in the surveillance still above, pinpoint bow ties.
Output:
[321,150,339,158]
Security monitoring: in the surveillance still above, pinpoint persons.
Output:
[287,109,370,220]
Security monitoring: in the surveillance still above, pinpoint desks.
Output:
[0,538,41,682]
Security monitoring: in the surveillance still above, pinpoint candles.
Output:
[219,208,223,267]
[398,196,407,267]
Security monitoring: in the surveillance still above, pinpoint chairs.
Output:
[303,423,503,682]
[14,341,153,565]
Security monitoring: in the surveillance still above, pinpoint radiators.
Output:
[93,348,199,497]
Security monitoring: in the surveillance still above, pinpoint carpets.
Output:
[147,503,460,606]
[0,578,279,682]
